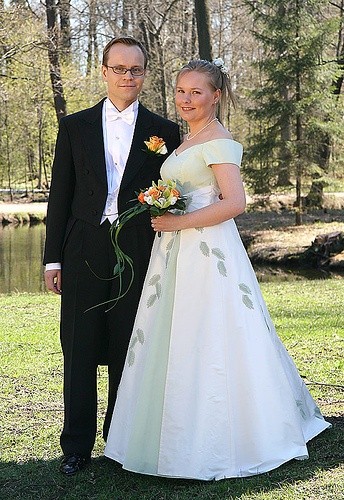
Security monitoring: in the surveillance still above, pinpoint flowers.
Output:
[212,56,230,73]
[144,135,168,155]
[129,180,187,236]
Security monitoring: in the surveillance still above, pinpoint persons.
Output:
[42,36,180,474]
[103,58,332,481]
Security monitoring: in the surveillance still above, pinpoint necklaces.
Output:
[186,118,222,140]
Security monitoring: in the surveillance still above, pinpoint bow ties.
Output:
[105,110,136,123]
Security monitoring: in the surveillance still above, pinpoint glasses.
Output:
[108,66,146,77]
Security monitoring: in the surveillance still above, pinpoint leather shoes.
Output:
[60,455,88,473]
